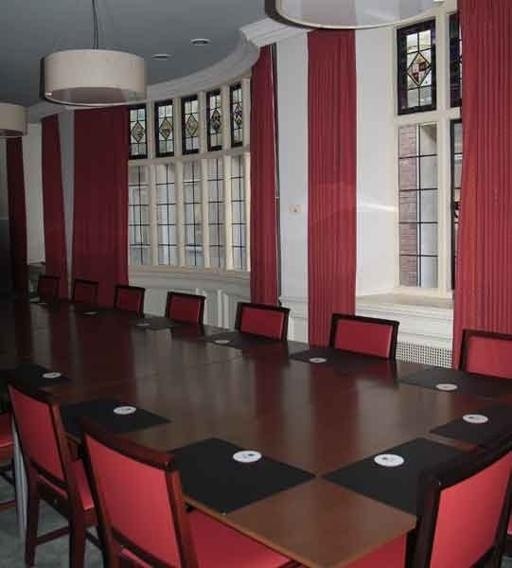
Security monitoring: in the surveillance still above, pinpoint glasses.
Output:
[0,97,30,140]
[43,1,149,110]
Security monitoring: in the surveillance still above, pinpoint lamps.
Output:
[43,1,149,110]
[0,97,30,140]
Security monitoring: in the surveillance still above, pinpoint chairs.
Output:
[235,302,290,341]
[112,283,145,313]
[165,291,205,324]
[76,414,300,567]
[0,407,17,508]
[412,432,507,568]
[6,381,99,567]
[458,329,509,378]
[37,275,60,300]
[328,313,400,361]
[71,278,99,305]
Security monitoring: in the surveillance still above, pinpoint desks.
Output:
[1,287,511,568]
